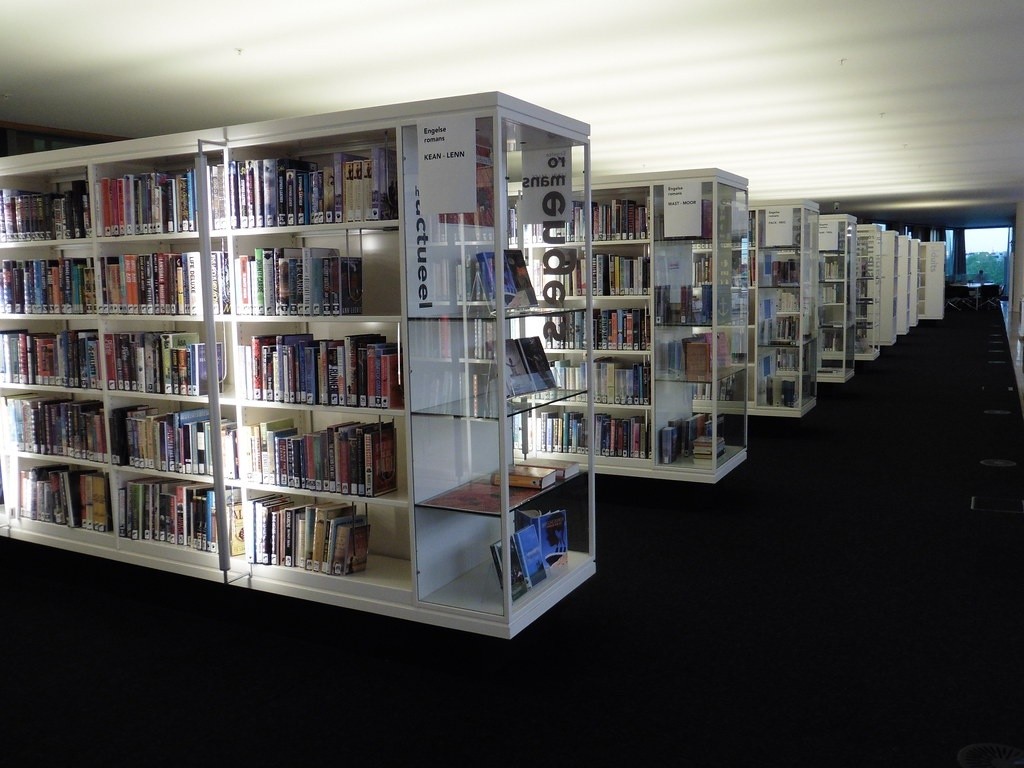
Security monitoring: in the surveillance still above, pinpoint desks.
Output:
[950,283,995,310]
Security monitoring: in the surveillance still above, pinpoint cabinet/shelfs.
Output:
[0,93,947,640]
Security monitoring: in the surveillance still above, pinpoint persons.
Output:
[975,270,985,283]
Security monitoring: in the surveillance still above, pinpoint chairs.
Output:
[945,281,1006,312]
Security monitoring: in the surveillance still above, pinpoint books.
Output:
[423,211,496,424]
[801,259,871,402]
[659,413,727,468]
[489,508,569,602]
[492,457,580,490]
[507,198,652,460]
[478,249,556,395]
[0,148,397,600]
[655,207,800,407]
